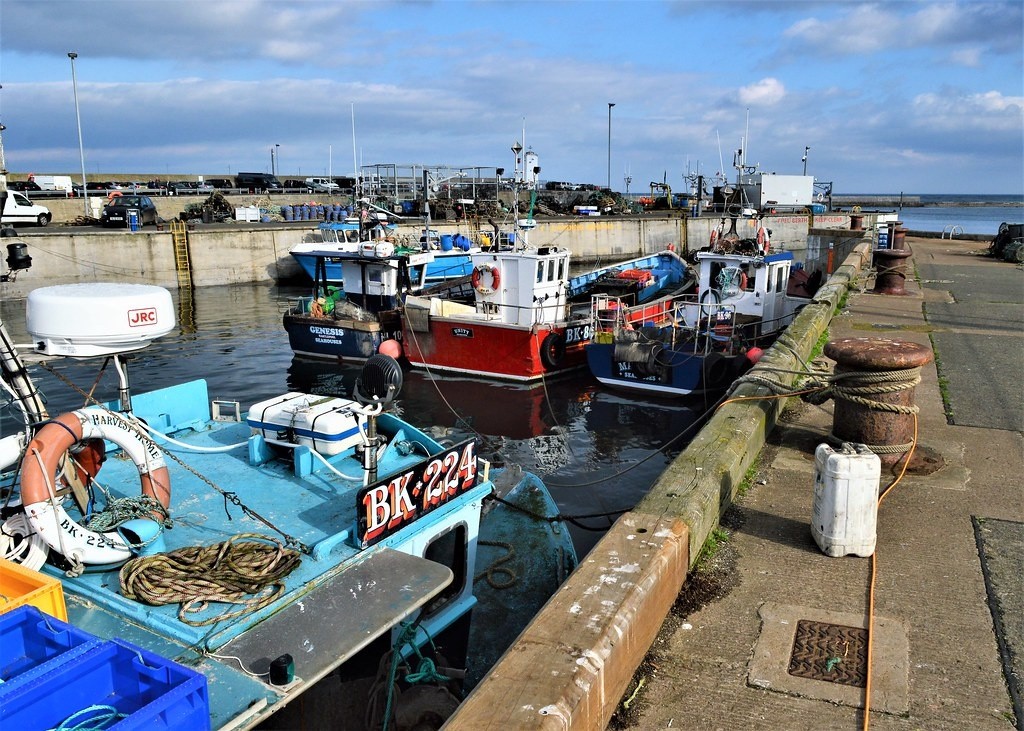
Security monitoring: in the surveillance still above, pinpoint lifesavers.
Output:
[758,227,770,256]
[20,407,170,565]
[472,263,501,295]
[109,191,124,206]
[710,230,716,247]
[718,267,747,291]
[540,333,568,372]
[28,173,34,183]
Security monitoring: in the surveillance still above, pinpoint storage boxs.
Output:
[616,270,651,283]
[0,604,101,697]
[0,637,212,731]
[715,324,732,336]
[0,556,67,624]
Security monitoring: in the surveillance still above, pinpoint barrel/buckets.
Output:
[461,236,472,250]
[280,203,353,221]
[452,234,463,247]
[814,443,881,557]
[441,235,453,250]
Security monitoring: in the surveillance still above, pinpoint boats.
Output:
[0,283,496,731]
[279,208,693,392]
[582,251,812,414]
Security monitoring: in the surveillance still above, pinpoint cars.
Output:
[555,181,587,191]
[0,190,52,226]
[74,179,232,197]
[284,177,340,194]
[12,181,42,198]
[101,195,158,228]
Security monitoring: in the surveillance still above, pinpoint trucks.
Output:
[28,176,73,196]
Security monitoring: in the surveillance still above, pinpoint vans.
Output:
[236,173,283,193]
